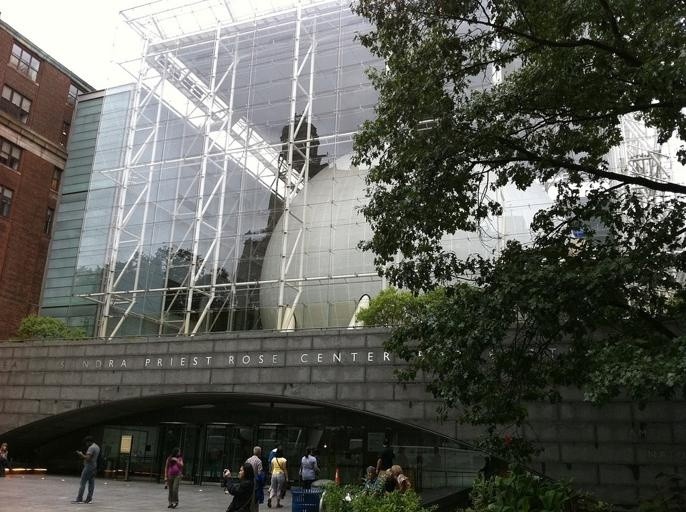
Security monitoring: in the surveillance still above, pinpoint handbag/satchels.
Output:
[284,480,291,490]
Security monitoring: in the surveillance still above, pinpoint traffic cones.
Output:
[334,467,340,486]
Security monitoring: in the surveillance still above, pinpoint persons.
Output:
[70,436,100,504]
[244,442,321,507]
[223,463,258,512]
[165,448,184,508]
[363,439,411,500]
[0,443,9,477]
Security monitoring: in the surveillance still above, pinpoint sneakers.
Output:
[268,498,272,507]
[167,503,177,508]
[277,505,283,508]
